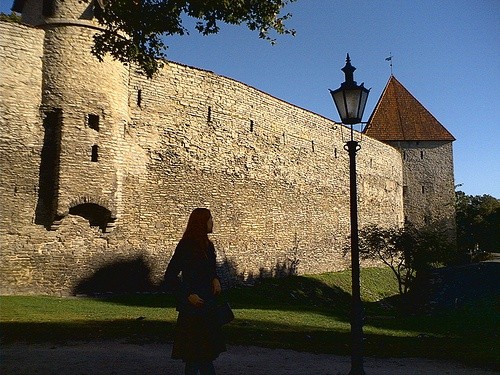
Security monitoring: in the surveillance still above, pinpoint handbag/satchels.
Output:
[205,294,233,326]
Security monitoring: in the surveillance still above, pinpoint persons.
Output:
[163,207,234,375]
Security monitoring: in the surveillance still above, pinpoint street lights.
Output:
[327,52,373,375]
